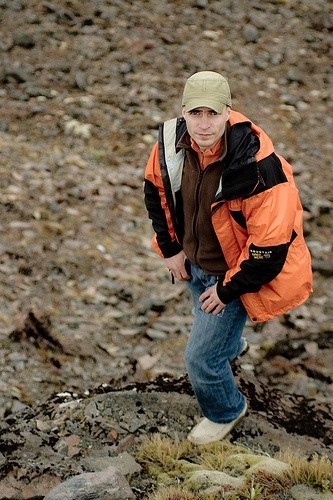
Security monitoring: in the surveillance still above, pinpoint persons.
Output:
[142,72,314,445]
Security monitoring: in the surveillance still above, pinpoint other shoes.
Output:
[228,337,250,363]
[187,394,248,445]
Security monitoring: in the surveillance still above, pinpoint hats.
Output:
[181,71,232,114]
